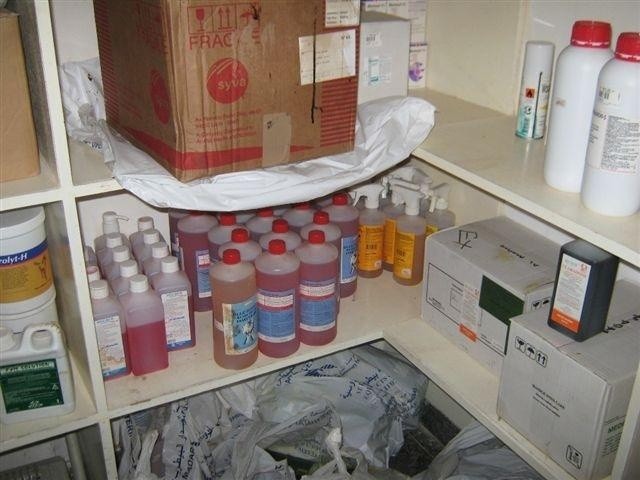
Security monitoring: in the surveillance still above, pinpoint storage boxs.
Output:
[1,11,38,183]
[495,278,640,480]
[91,2,363,187]
[421,212,571,376]
[359,3,431,103]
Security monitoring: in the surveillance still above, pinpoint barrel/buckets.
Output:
[0,206,76,424]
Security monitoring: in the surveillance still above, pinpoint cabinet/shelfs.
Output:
[385,112,640,480]
[1,3,533,480]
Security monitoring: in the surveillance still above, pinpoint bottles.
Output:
[516,21,640,218]
[82,189,456,381]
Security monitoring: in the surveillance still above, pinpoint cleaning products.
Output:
[82,185,455,382]
[543,20,640,214]
